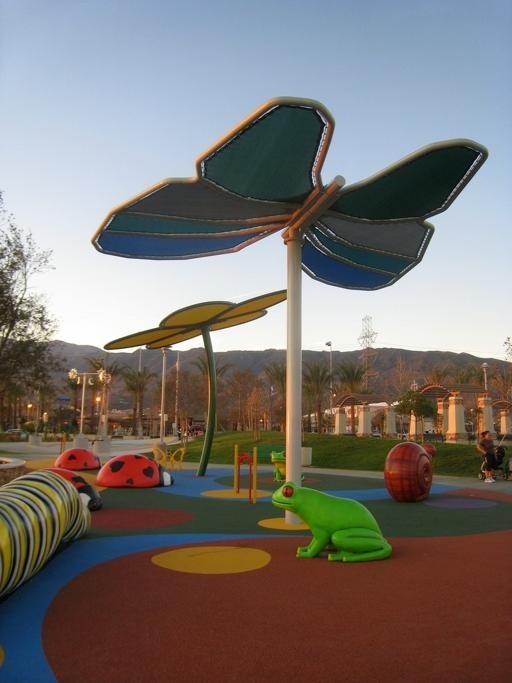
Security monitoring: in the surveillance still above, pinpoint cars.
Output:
[6,427,28,443]
[189,424,204,437]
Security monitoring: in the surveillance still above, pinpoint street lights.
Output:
[66,365,112,450]
[480,360,489,397]
[26,402,32,420]
[325,340,335,414]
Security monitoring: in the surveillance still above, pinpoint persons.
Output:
[476,430,497,483]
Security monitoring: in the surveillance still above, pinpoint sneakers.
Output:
[484,478,496,483]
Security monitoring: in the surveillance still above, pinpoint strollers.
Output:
[478,444,512,482]
